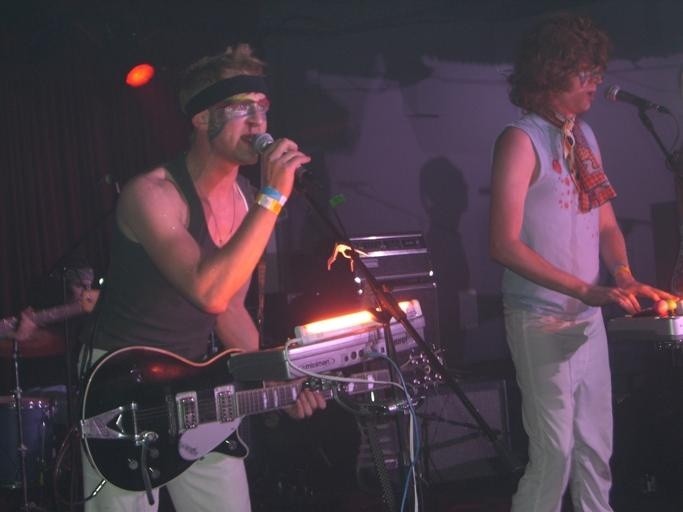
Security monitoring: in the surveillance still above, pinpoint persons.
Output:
[80,40,329,512]
[485,5,683,512]
[0,232,101,395]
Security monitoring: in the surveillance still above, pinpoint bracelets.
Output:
[253,185,288,206]
[250,193,282,216]
[610,263,632,278]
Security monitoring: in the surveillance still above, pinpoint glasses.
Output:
[572,66,607,80]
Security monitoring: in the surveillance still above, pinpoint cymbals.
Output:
[0,327,63,358]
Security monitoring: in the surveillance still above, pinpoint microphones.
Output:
[251,133,307,184]
[605,86,670,114]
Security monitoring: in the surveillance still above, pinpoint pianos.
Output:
[227,296,426,383]
[608,296,683,344]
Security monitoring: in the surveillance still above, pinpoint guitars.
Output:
[75,344,447,493]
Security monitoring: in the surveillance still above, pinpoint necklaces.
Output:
[192,170,237,248]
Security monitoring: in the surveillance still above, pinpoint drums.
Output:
[1,391,68,493]
